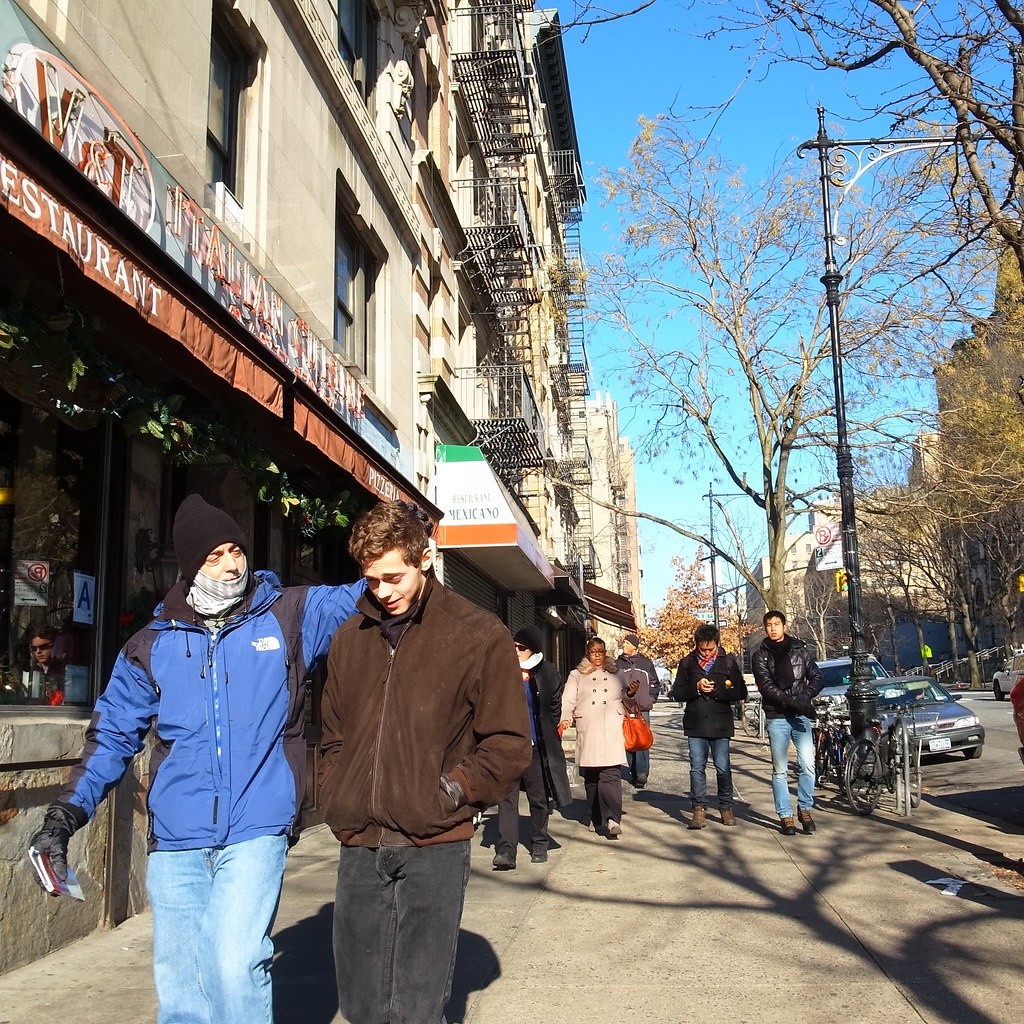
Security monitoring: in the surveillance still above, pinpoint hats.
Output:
[514,625,542,654]
[625,634,639,649]
[174,494,250,588]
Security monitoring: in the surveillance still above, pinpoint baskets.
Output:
[885,711,940,738]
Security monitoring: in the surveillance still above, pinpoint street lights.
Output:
[700,482,794,647]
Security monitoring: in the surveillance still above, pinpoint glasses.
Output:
[588,649,607,655]
[515,642,529,652]
[30,643,54,652]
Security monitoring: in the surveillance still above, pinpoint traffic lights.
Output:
[839,573,848,591]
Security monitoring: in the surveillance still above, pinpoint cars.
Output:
[814,654,903,726]
[870,676,986,767]
[745,683,760,699]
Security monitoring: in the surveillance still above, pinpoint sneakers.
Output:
[797,806,816,831]
[530,849,547,863]
[689,806,707,828]
[720,809,736,825]
[780,816,796,835]
[492,852,516,870]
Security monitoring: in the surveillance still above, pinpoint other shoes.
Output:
[607,818,623,835]
[589,821,603,832]
[635,774,647,788]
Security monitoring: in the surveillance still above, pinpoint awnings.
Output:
[0,97,444,530]
[549,564,640,637]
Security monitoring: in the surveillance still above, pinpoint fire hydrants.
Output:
[735,700,746,720]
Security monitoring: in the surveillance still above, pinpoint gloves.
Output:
[394,497,434,537]
[795,701,817,720]
[795,692,808,704]
[27,803,90,897]
[706,683,720,699]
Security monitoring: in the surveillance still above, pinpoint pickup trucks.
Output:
[993,649,1024,701]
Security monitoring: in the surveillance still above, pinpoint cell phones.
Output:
[626,680,640,691]
[708,682,715,685]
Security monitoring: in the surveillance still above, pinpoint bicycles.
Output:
[742,697,762,737]
[811,700,860,794]
[843,701,924,817]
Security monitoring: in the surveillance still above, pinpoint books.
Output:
[28,849,85,903]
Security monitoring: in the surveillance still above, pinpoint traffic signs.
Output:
[705,619,727,628]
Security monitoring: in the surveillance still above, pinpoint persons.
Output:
[673,624,748,829]
[904,689,923,706]
[26,624,64,706]
[613,634,660,788]
[26,494,432,1024]
[490,625,573,870]
[315,501,532,1023]
[752,611,825,834]
[561,637,639,835]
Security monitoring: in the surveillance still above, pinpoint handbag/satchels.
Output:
[622,700,654,750]
